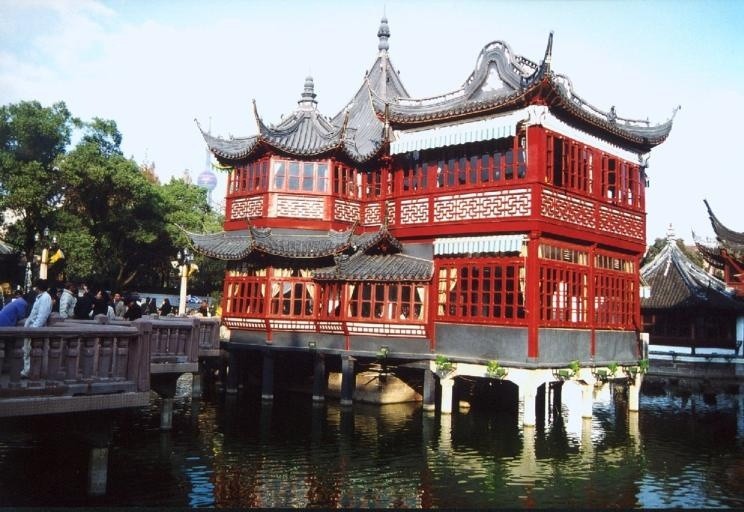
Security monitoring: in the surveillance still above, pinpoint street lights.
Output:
[171,247,200,317]
[34,227,65,292]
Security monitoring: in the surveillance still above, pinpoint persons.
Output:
[0,278,223,378]
